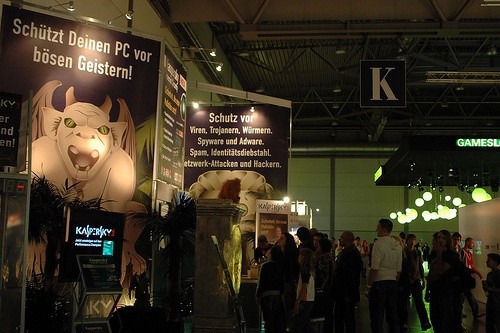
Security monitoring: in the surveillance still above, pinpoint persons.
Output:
[364,219,403,333]
[254,218,500,333]
[327,231,362,333]
[482,253,500,333]
[291,248,316,333]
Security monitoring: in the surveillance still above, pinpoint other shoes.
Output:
[421,323,432,331]
[462,313,468,318]
[474,312,485,319]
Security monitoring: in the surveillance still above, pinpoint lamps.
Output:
[108,9,134,24]
[49,1,75,12]
[182,59,223,72]
[172,46,216,57]
